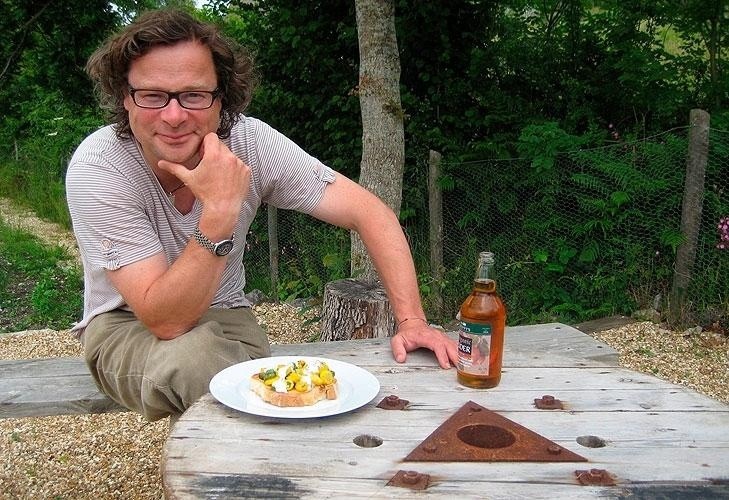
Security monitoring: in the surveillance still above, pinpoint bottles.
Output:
[454,250,507,390]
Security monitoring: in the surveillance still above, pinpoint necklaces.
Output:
[162,181,185,207]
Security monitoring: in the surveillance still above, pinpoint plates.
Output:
[207,354,382,420]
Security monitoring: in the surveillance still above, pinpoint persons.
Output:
[62,5,458,424]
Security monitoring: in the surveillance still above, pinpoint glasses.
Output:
[126,82,221,110]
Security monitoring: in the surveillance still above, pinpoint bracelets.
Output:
[395,317,428,328]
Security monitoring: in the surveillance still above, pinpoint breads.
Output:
[250,371,339,406]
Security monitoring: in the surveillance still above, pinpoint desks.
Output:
[158,347,729,500]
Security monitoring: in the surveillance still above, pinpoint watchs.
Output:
[195,222,236,257]
